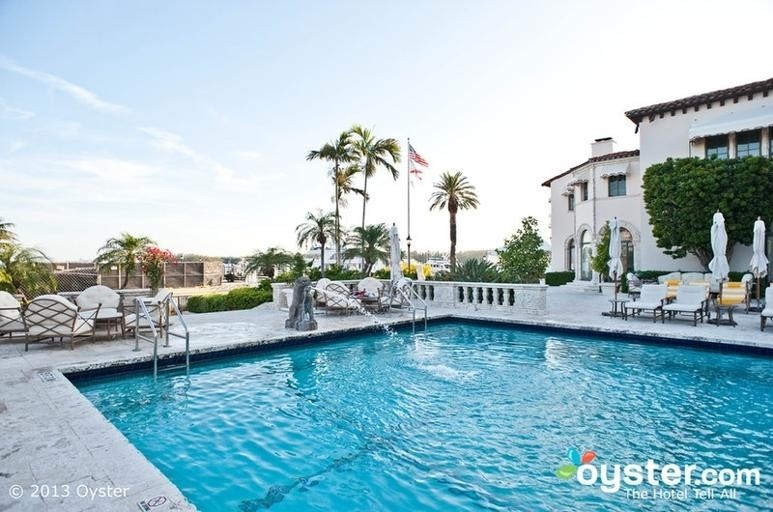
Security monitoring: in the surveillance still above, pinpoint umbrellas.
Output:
[750,216,769,299]
[709,210,730,305]
[389,222,403,279]
[607,216,624,314]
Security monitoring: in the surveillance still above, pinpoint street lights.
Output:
[404,235,413,278]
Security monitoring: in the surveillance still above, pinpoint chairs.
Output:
[279,277,414,317]
[0,285,174,351]
[608,272,773,331]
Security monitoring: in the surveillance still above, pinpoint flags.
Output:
[408,143,430,181]
